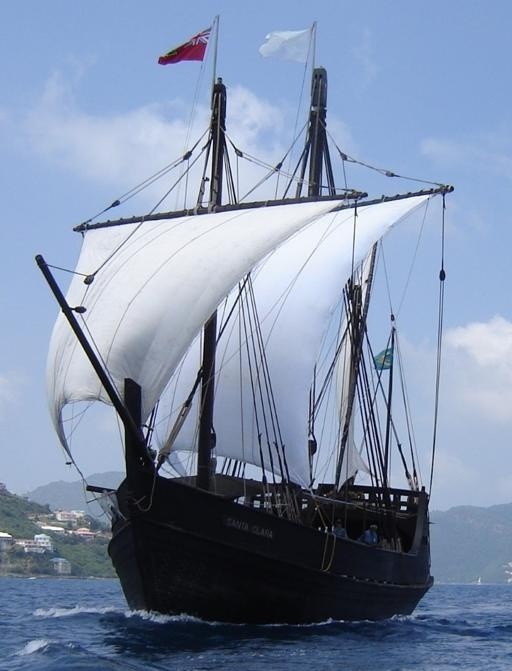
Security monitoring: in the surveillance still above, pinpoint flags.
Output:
[157,25,213,65]
[374,347,393,370]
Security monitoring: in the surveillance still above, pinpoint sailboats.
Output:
[35,15,455,628]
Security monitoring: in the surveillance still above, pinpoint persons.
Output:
[357,524,385,547]
[333,517,347,537]
